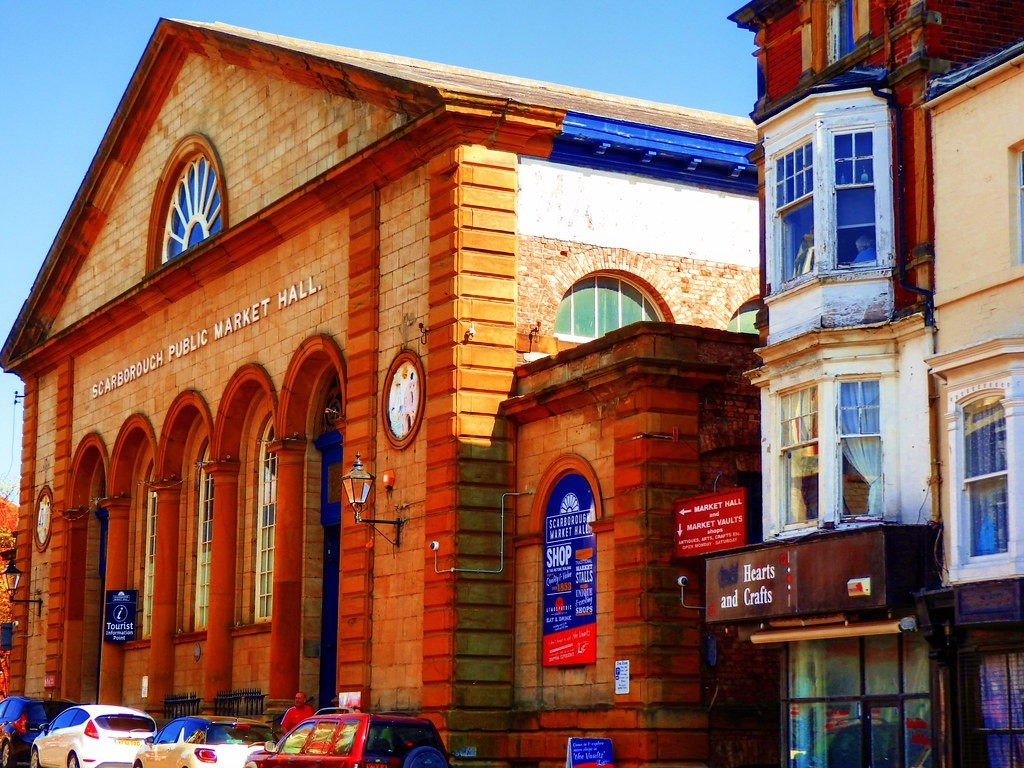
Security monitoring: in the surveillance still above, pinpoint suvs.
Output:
[244,707,454,768]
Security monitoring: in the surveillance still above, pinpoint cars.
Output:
[0,694,79,768]
[133,715,277,767]
[27,704,157,768]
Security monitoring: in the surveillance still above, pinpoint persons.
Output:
[852,234,876,262]
[279,691,314,736]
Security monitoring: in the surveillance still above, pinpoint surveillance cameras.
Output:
[430,541,438,550]
[13,621,19,627]
[677,576,688,586]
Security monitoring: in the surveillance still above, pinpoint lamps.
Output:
[2,560,43,617]
[341,450,403,547]
